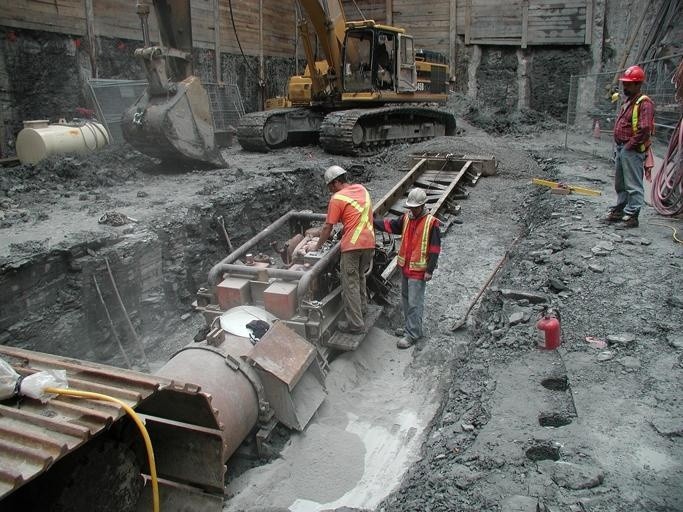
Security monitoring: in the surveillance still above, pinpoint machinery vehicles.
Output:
[0,339,228,512]
[113,0,464,169]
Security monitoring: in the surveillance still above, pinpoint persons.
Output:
[305,165,376,334]
[372,187,441,349]
[611,92,619,108]
[605,66,656,231]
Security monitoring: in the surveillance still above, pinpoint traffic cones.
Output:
[589,120,603,143]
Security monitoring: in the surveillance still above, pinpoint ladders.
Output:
[93,257,151,373]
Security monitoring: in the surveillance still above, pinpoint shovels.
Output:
[450,237,518,331]
[217,215,246,265]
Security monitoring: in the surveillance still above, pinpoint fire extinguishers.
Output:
[536,305,561,350]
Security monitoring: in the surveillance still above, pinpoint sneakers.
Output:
[397,335,413,349]
[337,320,363,334]
[615,215,640,230]
[394,327,406,336]
[603,208,622,221]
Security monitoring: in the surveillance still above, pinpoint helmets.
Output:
[610,93,619,104]
[406,187,427,208]
[323,165,346,185]
[618,65,646,83]
